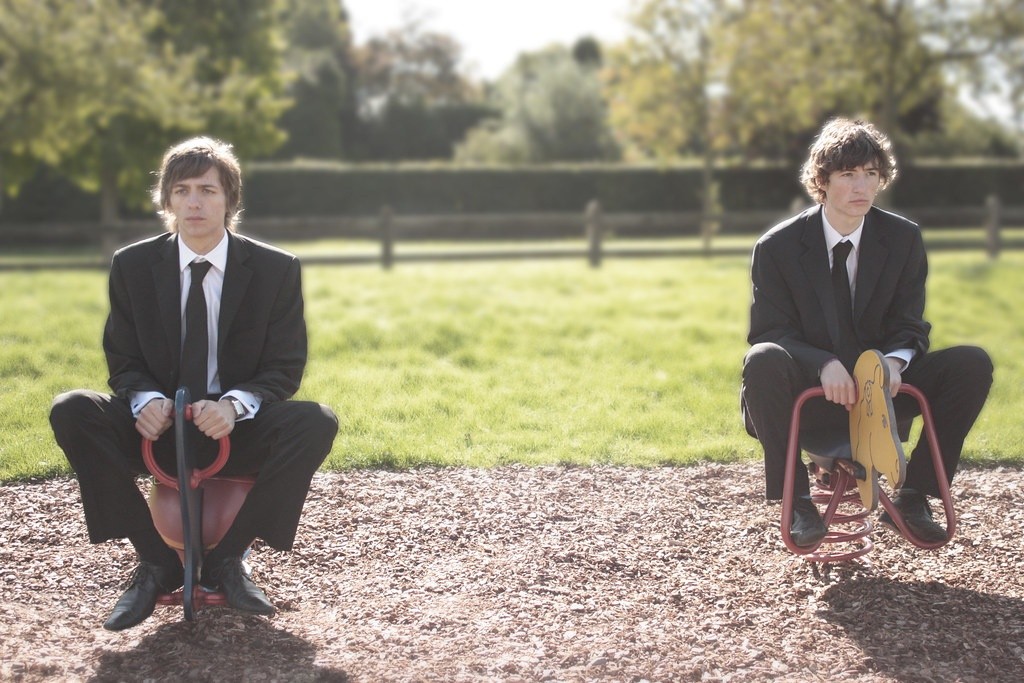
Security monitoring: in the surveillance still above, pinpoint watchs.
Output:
[222,396,247,420]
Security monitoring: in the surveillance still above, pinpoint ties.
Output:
[831,240,853,307]
[181,260,213,402]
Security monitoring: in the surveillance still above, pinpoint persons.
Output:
[741,114,995,547]
[49,136,339,631]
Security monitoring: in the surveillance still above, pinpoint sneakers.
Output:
[790,497,828,547]
[877,490,949,542]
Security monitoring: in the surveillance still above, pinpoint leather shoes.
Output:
[201,553,276,615]
[104,548,186,632]
